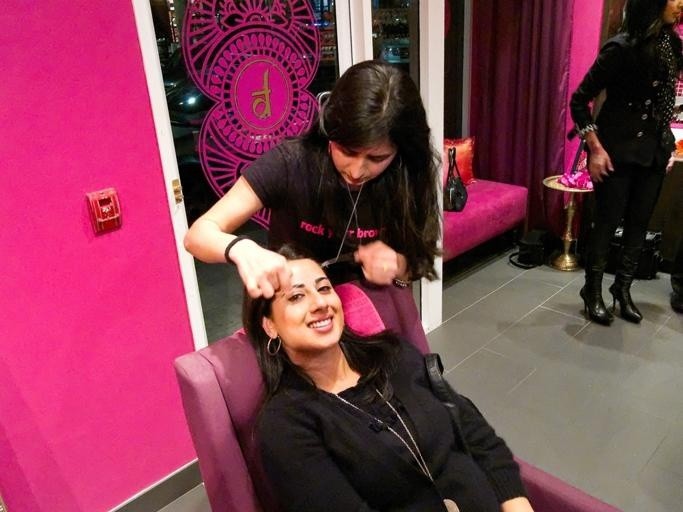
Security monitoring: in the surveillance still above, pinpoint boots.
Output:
[579,244,615,327]
[609,240,643,324]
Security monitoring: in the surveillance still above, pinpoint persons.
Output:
[184,61,444,288]
[569,0,683,326]
[242,244,534,512]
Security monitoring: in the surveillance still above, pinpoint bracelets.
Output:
[225,235,251,266]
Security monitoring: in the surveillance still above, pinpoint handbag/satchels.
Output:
[441,145,469,214]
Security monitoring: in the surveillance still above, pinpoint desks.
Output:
[542,171,596,269]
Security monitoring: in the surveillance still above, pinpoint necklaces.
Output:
[312,383,460,512]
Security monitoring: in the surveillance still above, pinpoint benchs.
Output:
[442,178,535,286]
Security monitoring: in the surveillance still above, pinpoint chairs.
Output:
[175,272,628,512]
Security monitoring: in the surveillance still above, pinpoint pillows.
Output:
[440,135,478,183]
